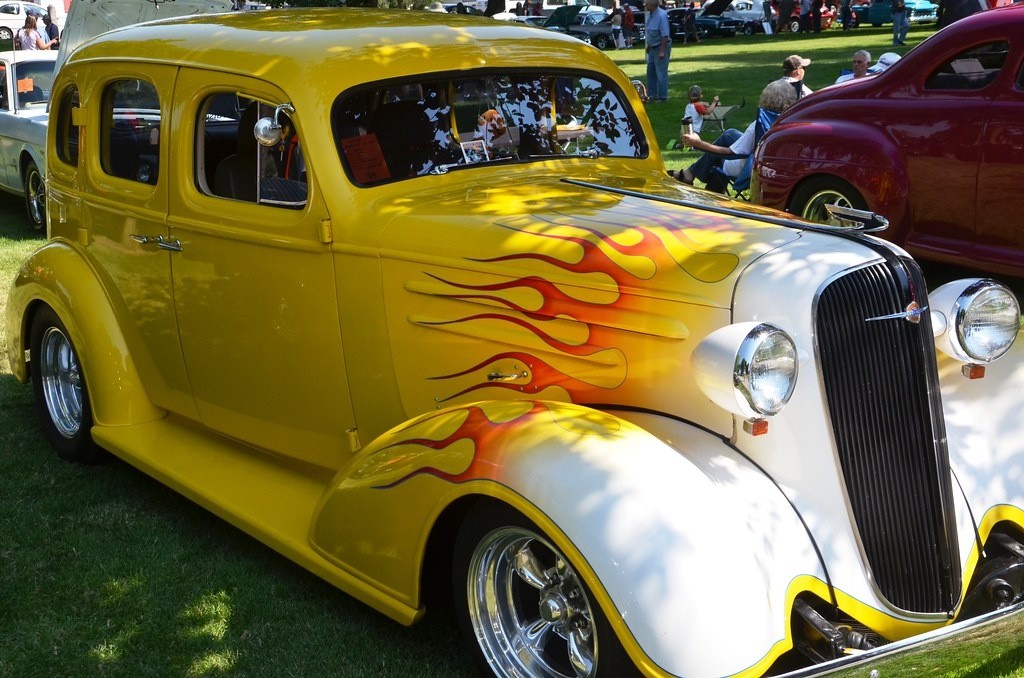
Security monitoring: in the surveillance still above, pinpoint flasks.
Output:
[682,117,693,147]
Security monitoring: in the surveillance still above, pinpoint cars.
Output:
[0,0,61,40]
[0,48,243,232]
[835,0,939,28]
[505,0,838,50]
[3,8,1024,678]
[749,2,1024,284]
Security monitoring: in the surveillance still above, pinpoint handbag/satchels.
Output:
[15,30,21,50]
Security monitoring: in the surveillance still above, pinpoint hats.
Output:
[783,54,811,71]
[41,15,50,20]
[867,53,902,73]
[689,85,702,100]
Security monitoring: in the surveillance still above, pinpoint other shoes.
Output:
[683,146,691,153]
[665,139,676,150]
[898,42,906,45]
[627,44,632,48]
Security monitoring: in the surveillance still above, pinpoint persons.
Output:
[15,9,59,49]
[611,3,634,50]
[842,0,870,31]
[645,0,671,103]
[799,0,837,34]
[779,54,815,98]
[427,0,543,16]
[891,0,910,47]
[763,0,795,37]
[834,50,872,83]
[683,2,704,45]
[665,85,719,152]
[276,3,283,9]
[867,52,902,73]
[213,100,279,202]
[667,80,797,194]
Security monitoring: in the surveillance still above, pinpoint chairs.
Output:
[698,96,745,134]
[213,101,258,202]
[359,100,452,185]
[705,107,782,202]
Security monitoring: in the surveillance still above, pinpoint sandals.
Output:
[666,169,694,185]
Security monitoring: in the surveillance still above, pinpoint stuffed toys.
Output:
[929,0,983,29]
